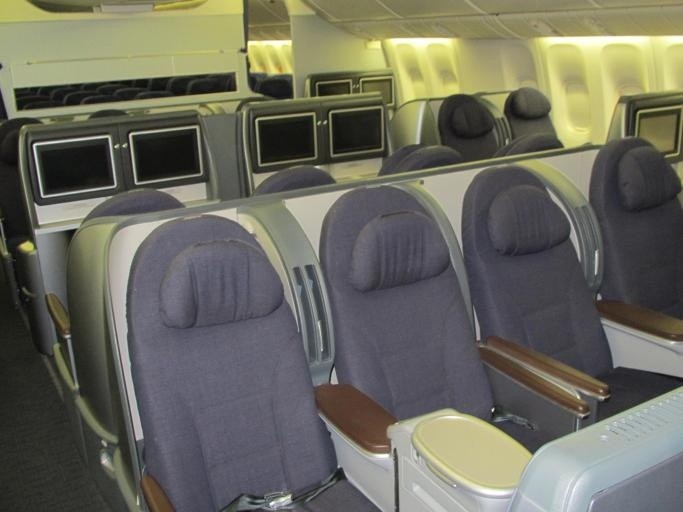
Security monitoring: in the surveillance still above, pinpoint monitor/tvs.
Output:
[254,112,318,166]
[358,75,395,106]
[129,124,203,186]
[316,79,352,96]
[32,134,117,199]
[328,105,385,158]
[634,104,683,158]
